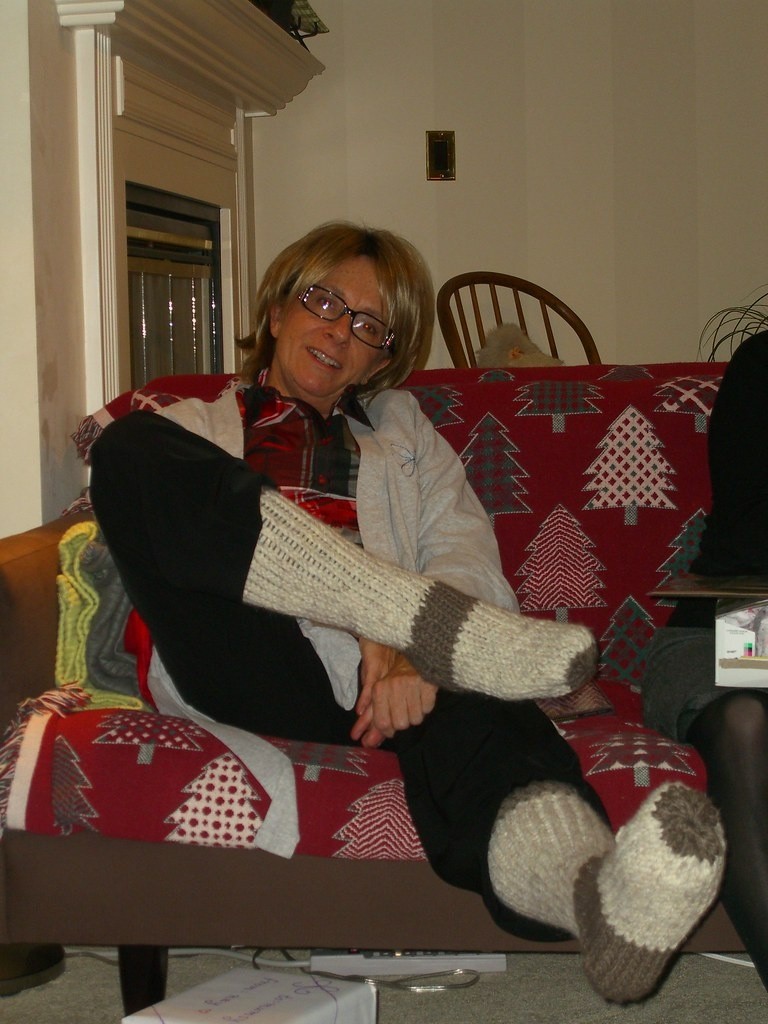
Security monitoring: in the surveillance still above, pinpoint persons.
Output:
[87,221,728,1007]
[641,330,768,989]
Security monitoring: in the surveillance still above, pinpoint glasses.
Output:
[292,284,396,352]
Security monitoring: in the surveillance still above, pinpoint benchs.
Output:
[0,364,727,1014]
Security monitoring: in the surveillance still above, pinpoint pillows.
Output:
[479,324,561,367]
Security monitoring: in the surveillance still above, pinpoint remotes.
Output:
[309,949,507,977]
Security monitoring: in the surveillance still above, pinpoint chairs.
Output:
[436,271,602,369]
